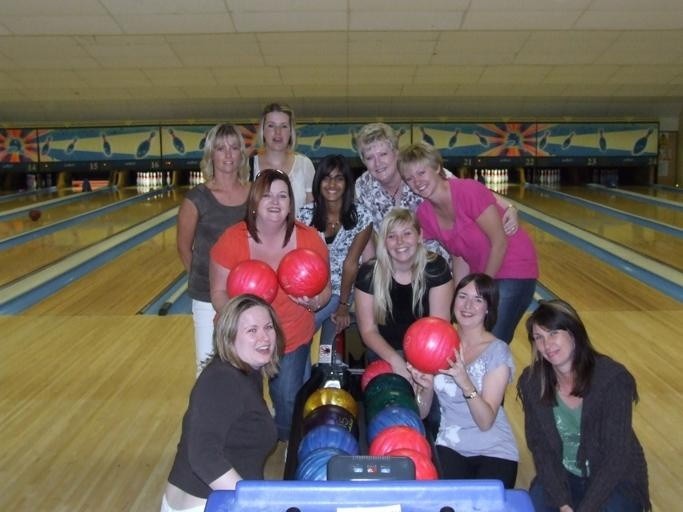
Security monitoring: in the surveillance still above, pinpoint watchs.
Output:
[462,386,478,400]
[504,204,518,211]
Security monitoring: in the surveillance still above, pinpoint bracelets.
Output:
[338,300,351,307]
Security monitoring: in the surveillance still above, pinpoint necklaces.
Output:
[389,184,400,206]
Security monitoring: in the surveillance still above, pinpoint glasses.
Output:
[256,168,286,177]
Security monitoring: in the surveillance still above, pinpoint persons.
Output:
[517,298,651,512]
[246,102,315,218]
[177,122,254,378]
[396,141,539,345]
[208,168,333,462]
[355,208,455,416]
[161,294,284,512]
[405,272,520,491]
[297,154,373,384]
[354,123,519,277]
